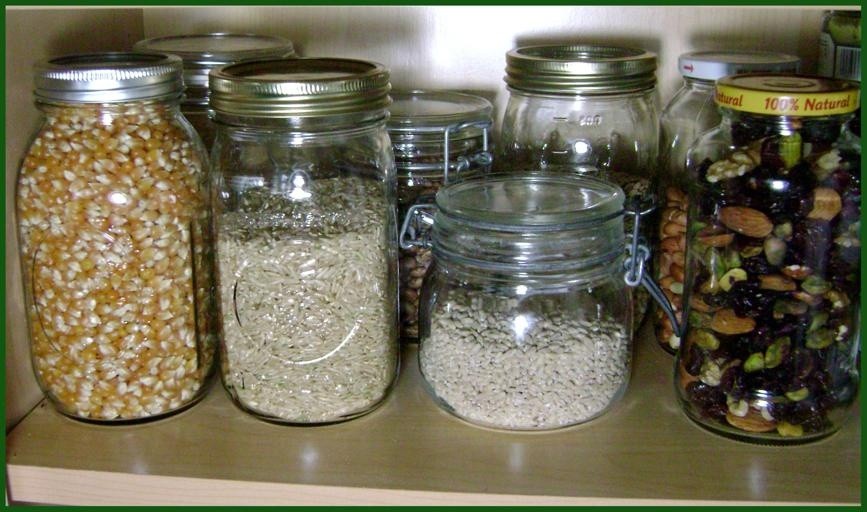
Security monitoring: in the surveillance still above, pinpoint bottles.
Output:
[661,50,803,361]
[402,173,640,430]
[136,32,297,117]
[367,91,495,340]
[674,48,864,444]
[503,44,663,338]
[16,51,213,423]
[212,57,397,427]
